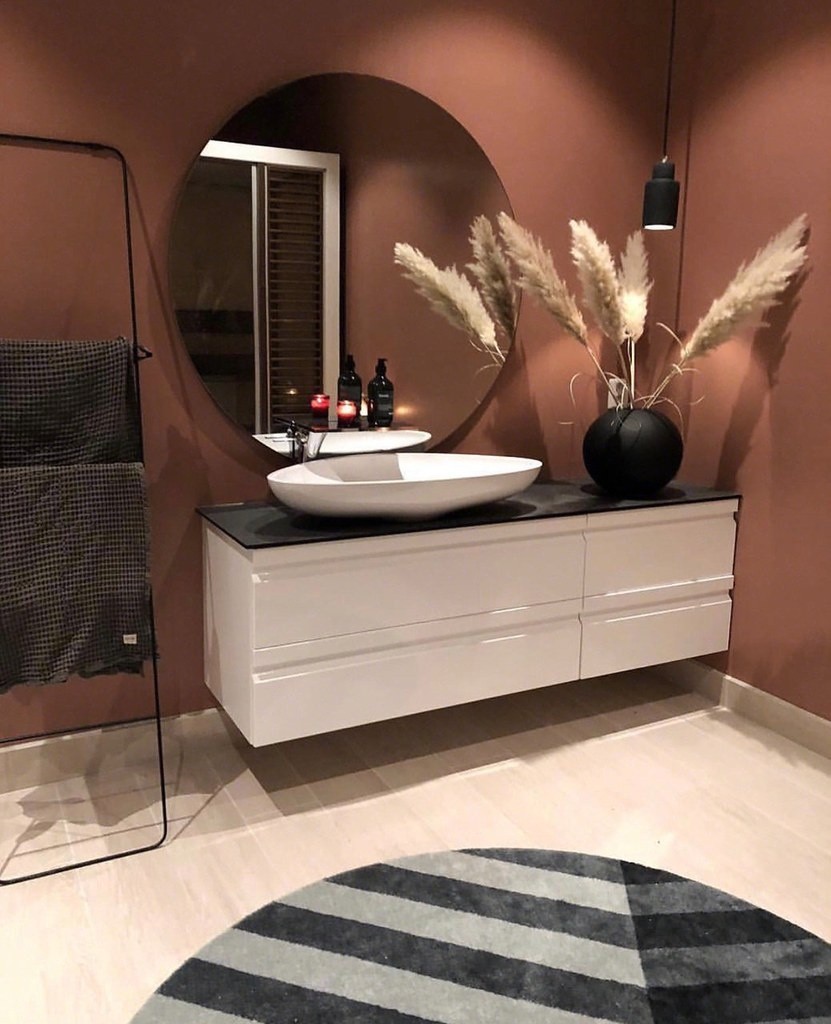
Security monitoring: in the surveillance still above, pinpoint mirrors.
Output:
[169,70,524,464]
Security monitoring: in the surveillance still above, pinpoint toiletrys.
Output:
[367,357,395,429]
[338,354,363,419]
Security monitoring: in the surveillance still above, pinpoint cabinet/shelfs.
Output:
[200,498,738,749]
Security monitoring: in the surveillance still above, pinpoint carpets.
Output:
[128,848,831,1024]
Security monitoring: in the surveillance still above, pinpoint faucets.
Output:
[272,424,309,462]
[264,417,295,457]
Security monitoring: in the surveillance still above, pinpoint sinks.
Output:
[265,453,544,518]
[251,429,432,456]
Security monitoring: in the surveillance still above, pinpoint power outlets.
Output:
[607,379,628,408]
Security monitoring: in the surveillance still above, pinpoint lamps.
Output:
[642,0,680,231]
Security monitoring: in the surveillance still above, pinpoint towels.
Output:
[0,336,161,694]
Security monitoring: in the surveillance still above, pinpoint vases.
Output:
[584,404,684,495]
